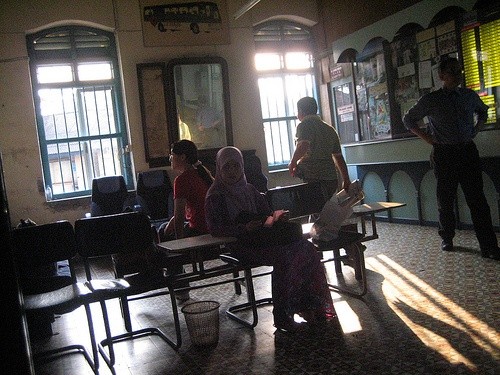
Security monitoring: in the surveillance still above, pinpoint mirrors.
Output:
[167,56,233,159]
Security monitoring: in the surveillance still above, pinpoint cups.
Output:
[86,213,91,218]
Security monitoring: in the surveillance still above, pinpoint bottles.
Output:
[46,185,52,201]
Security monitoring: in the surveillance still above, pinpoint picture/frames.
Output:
[136,62,173,163]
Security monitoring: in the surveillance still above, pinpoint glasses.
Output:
[169,154,174,156]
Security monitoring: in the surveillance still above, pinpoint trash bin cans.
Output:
[181,300,220,348]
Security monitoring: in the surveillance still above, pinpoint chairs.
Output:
[7,155,367,375]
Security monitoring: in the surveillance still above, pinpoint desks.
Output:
[352,202,407,235]
[340,128,500,232]
[156,234,239,276]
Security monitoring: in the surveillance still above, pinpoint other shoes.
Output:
[482,247,500,260]
[441,238,453,250]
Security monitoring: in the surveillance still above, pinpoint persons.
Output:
[177,94,225,148]
[402,56,500,261]
[204,147,343,335]
[158,139,216,312]
[287,97,368,263]
